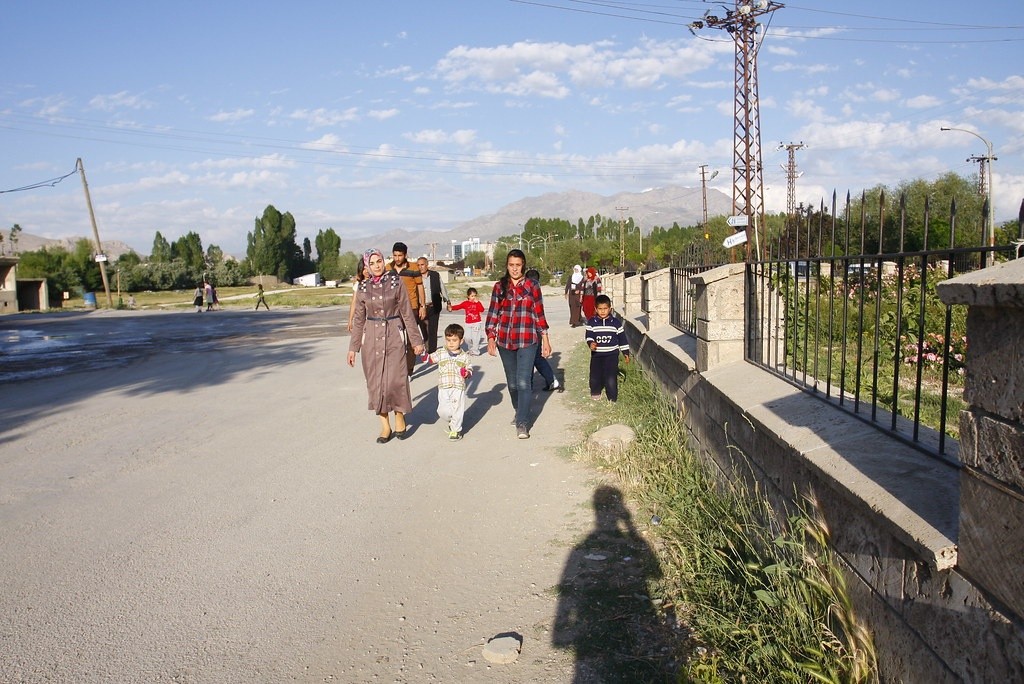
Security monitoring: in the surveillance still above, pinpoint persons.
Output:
[204,281,220,311]
[115,294,124,306]
[384,242,428,383]
[128,294,136,305]
[346,248,424,442]
[485,249,562,437]
[193,285,204,313]
[565,264,585,329]
[575,267,602,321]
[253,284,270,311]
[447,287,485,356]
[417,256,452,355]
[420,323,474,442]
[586,295,630,402]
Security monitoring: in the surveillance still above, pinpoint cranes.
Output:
[425,241,440,258]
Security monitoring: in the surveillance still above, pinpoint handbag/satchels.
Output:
[399,325,424,346]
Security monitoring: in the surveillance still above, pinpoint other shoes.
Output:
[377,431,392,443]
[543,381,560,391]
[592,394,601,400]
[516,424,529,439]
[395,419,406,437]
[510,419,517,426]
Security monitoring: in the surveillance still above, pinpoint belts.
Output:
[367,315,400,321]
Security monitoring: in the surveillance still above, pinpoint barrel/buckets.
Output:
[83,292,96,306]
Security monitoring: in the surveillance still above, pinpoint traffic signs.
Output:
[723,230,748,248]
[726,215,748,226]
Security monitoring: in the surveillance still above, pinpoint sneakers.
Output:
[448,432,462,441]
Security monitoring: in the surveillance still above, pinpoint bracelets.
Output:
[446,302,451,305]
[419,304,427,309]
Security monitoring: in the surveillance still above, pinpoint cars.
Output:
[847,263,878,275]
[556,271,564,276]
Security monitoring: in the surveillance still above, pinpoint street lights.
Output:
[517,236,541,253]
[940,126,995,264]
[518,223,522,250]
[531,233,559,254]
